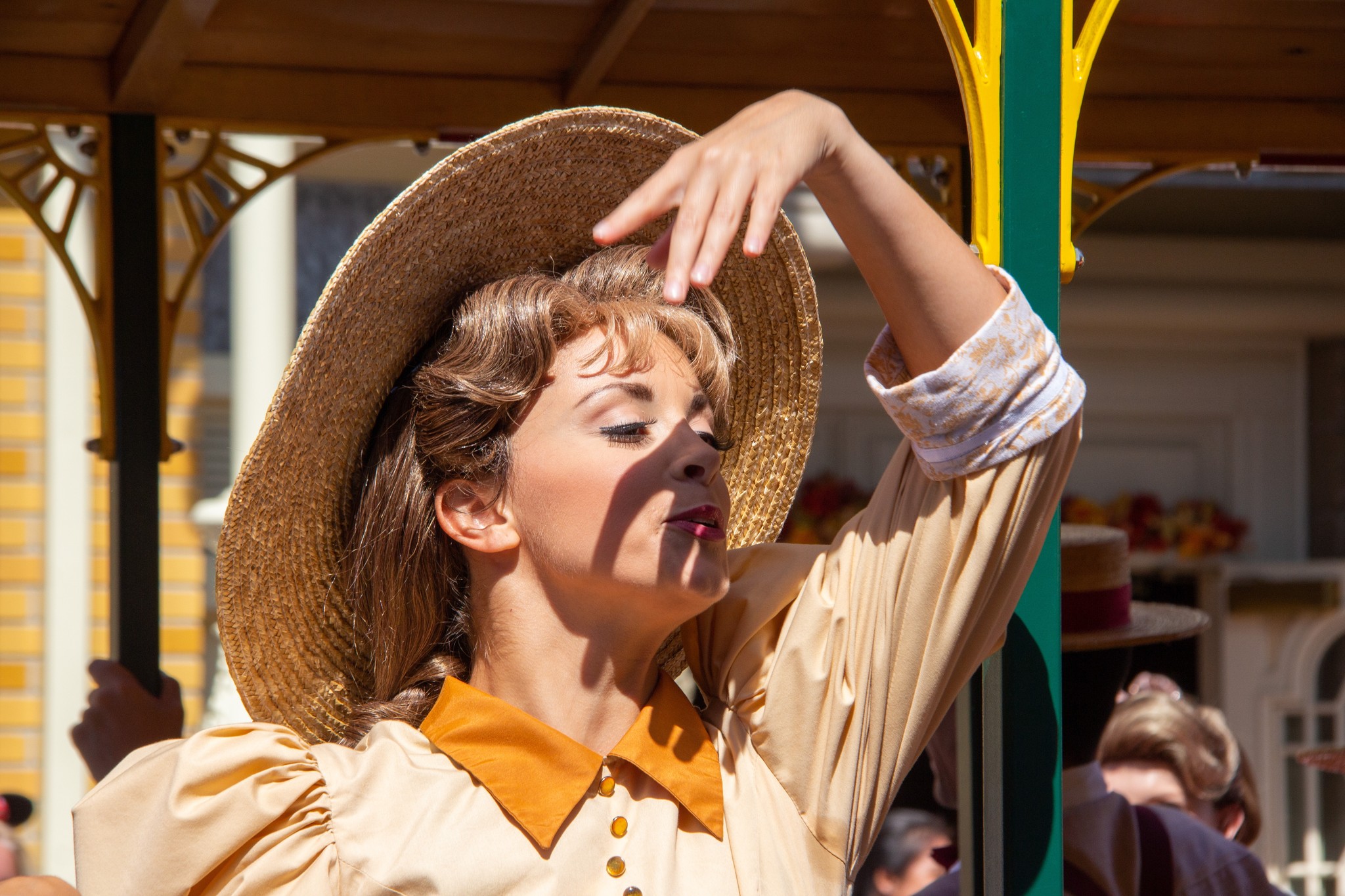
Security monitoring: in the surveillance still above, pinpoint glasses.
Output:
[928,843,960,873]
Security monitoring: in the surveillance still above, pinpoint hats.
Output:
[1062,517,1211,654]
[211,105,824,749]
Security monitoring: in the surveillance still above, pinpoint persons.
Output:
[906,525,1291,896]
[850,810,960,896]
[72,88,1084,896]
[0,654,183,896]
[1091,671,1259,857]
